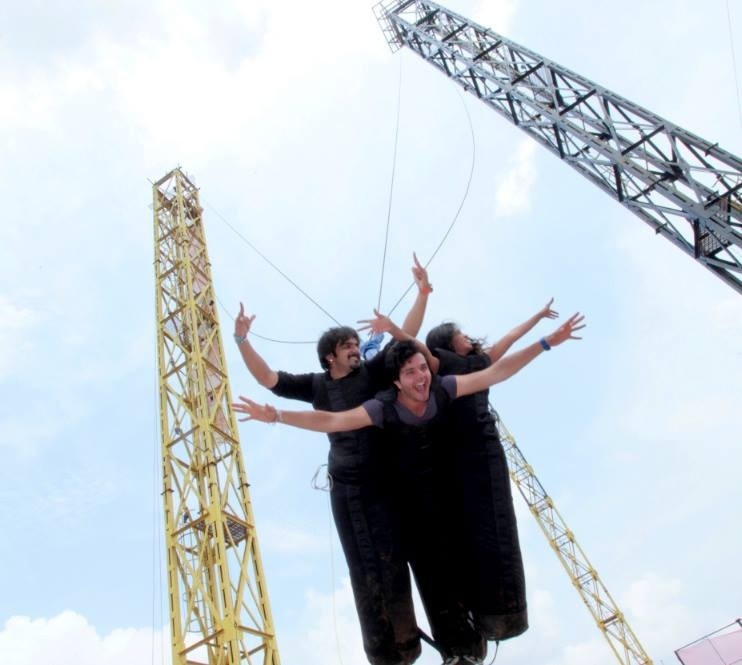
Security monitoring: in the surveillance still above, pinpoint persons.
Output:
[356,297,559,641]
[229,313,588,665]
[232,250,433,665]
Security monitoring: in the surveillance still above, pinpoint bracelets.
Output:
[540,336,551,351]
[233,335,248,344]
[273,407,282,424]
[420,287,433,293]
